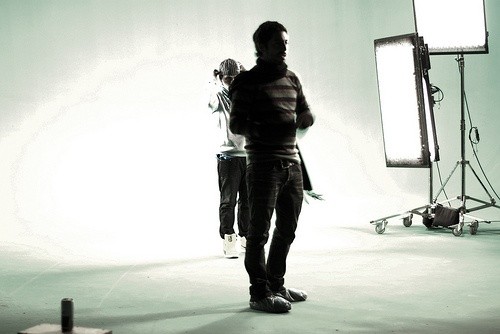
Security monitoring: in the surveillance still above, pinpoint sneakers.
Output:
[236,235,247,252]
[223,234,239,258]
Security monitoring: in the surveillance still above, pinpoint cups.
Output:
[61,298,73,334]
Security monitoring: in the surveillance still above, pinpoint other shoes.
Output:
[276,288,308,302]
[250,294,292,314]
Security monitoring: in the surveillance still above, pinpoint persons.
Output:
[227,21,314,313]
[205,59,248,258]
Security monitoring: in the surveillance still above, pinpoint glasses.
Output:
[222,75,237,83]
